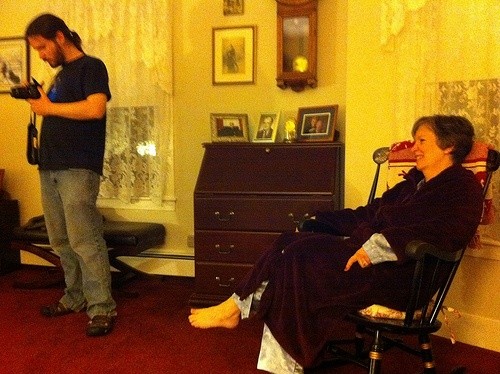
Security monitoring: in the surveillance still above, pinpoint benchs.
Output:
[9,220,167,298]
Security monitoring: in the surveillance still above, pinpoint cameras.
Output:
[10,78,42,98]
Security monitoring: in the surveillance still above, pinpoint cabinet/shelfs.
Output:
[188,141,341,307]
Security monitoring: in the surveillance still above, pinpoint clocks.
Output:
[275,0,318,92]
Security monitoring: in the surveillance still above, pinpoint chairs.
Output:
[307,139,500,374]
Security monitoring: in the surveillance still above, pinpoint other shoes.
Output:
[85,313,117,337]
[40,300,73,317]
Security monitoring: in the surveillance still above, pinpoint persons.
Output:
[257,116,273,138]
[19,14,117,336]
[188,114,483,374]
[304,117,326,133]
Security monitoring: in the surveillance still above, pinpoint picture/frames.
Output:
[212,24,255,85]
[209,112,249,143]
[0,36,30,95]
[253,110,282,143]
[296,104,338,141]
